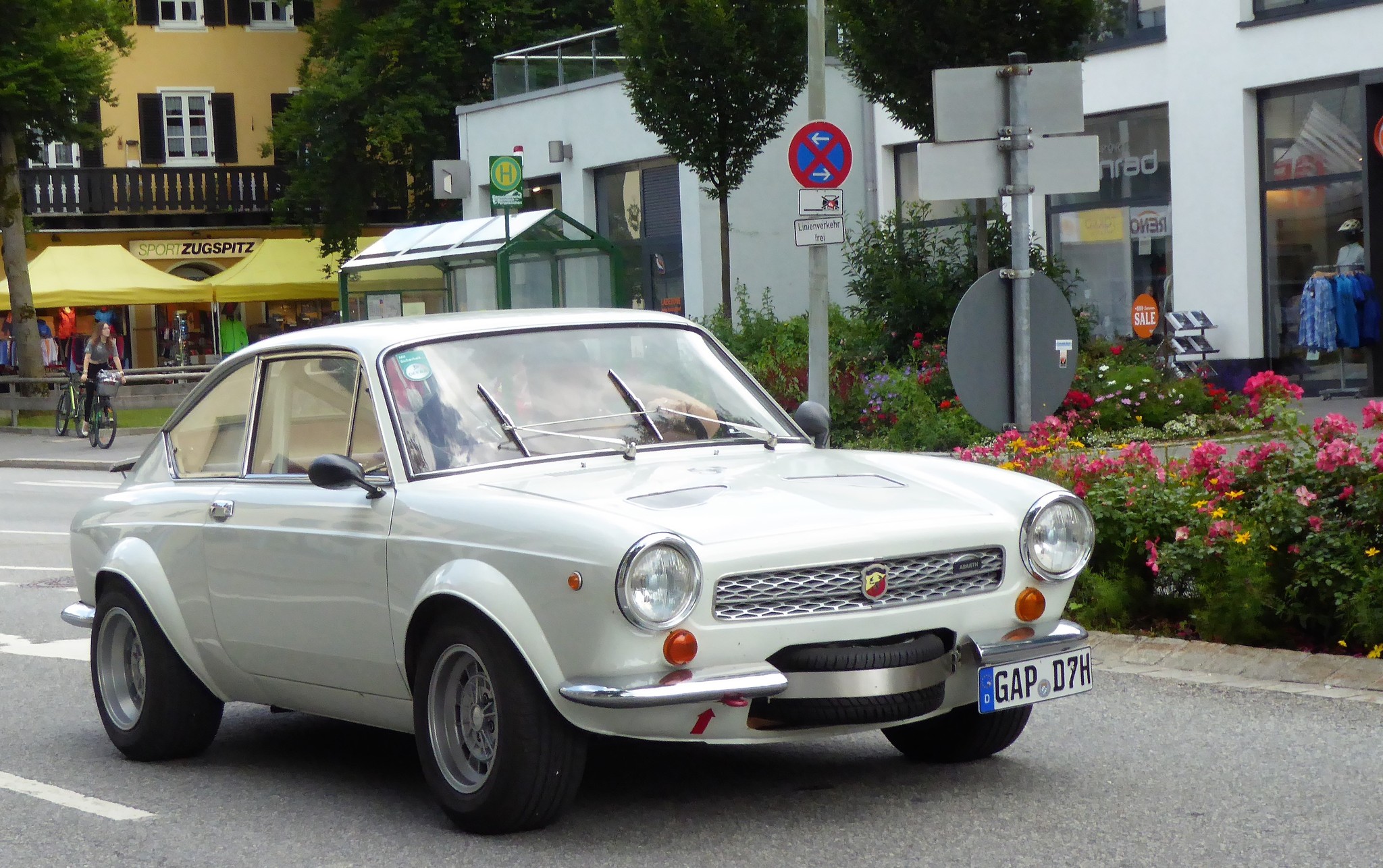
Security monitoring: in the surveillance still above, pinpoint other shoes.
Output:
[102,418,114,423]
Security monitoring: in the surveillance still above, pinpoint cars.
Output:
[56,306,1102,839]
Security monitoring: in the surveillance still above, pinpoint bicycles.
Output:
[80,374,123,449]
[55,369,97,438]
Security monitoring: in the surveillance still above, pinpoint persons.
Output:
[58,306,77,355]
[95,305,116,337]
[80,322,126,436]
[1336,219,1364,272]
[523,338,720,441]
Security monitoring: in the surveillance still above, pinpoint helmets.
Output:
[1338,219,1361,233]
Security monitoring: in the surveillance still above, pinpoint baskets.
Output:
[96,378,120,397]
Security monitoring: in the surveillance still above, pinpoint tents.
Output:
[200,236,443,359]
[0,245,216,356]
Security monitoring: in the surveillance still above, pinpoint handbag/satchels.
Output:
[82,422,89,437]
